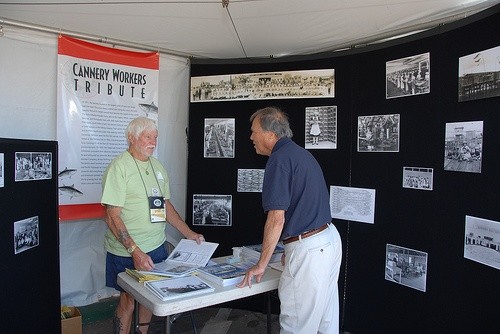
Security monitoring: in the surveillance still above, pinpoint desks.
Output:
[117,254,284,334]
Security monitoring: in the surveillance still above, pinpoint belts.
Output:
[282,223,330,245]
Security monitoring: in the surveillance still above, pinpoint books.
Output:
[141,239,219,277]
[233,243,285,263]
[195,257,262,287]
[126,267,214,300]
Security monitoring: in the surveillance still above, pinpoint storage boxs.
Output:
[61,306,83,334]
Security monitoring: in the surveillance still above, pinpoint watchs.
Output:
[128,245,138,254]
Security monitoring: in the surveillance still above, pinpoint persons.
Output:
[101,116,205,334]
[237,106,343,334]
[448,144,480,162]
[34,156,49,168]
[310,116,322,145]
[16,226,38,247]
